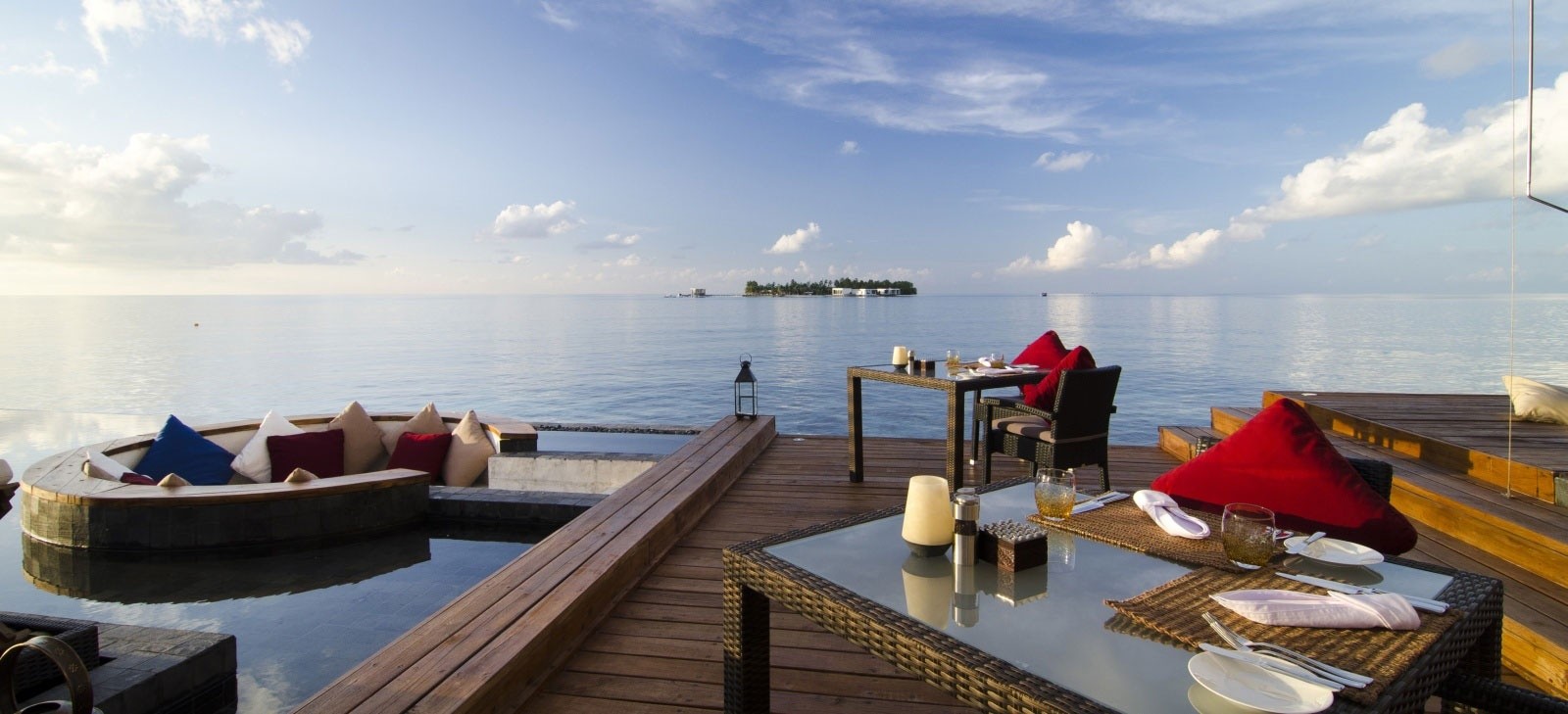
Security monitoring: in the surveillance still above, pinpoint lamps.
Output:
[734,352,758,420]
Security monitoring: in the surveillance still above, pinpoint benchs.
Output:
[21,415,538,541]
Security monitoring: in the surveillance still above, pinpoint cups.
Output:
[1221,503,1275,569]
[947,349,960,365]
[990,353,1004,369]
[1035,467,1077,521]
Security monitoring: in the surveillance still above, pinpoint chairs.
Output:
[1429,670,1568,714]
[970,350,1075,466]
[1196,435,1391,502]
[983,365,1121,491]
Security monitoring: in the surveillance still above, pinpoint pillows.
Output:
[83,402,495,487]
[1503,375,1568,424]
[1149,397,1417,558]
[1011,332,1098,425]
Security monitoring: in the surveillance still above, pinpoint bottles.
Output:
[952,487,980,566]
[907,350,915,369]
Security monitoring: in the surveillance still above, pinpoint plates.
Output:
[958,372,985,379]
[1283,535,1384,567]
[1011,364,1039,369]
[1187,651,1334,714]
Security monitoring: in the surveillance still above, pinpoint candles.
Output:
[892,346,908,365]
[902,476,954,545]
[901,568,953,626]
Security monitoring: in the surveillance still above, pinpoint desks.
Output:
[848,359,1055,492]
[723,474,1502,714]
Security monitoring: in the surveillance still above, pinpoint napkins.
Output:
[970,356,1024,374]
[1212,591,1420,632]
[1133,489,1211,540]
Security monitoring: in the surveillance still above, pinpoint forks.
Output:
[1202,611,1374,689]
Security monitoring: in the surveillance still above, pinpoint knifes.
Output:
[1284,532,1326,555]
[1199,642,1345,691]
